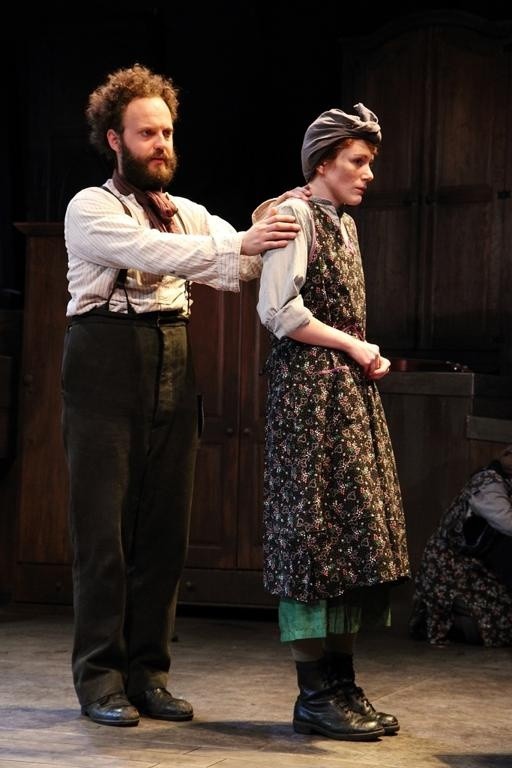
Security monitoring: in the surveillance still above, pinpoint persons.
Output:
[256,99,411,741]
[407,442,510,648]
[60,58,316,725]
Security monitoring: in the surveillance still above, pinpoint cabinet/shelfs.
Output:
[330,50,509,359]
[14,221,364,624]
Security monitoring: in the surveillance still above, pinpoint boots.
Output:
[289,651,402,743]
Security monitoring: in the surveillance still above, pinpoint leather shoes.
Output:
[81,683,197,727]
